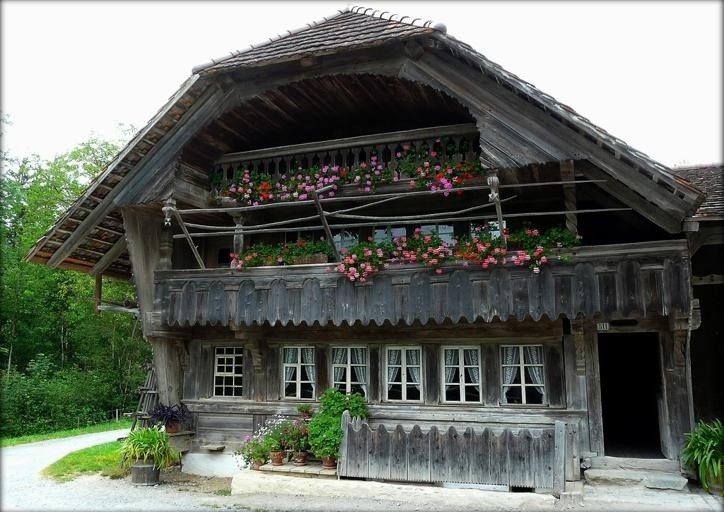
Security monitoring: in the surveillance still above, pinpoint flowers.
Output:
[232,411,310,472]
[391,227,458,264]
[526,228,539,237]
[278,257,283,262]
[207,138,490,206]
[320,237,324,240]
[333,237,389,282]
[231,253,257,270]
[463,228,510,269]
[281,243,288,250]
[510,246,550,274]
[229,217,582,283]
[267,256,272,260]
[298,239,305,246]
[436,268,443,275]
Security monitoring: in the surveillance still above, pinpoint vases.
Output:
[291,254,328,264]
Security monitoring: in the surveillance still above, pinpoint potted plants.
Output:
[679,418,724,496]
[116,402,191,486]
[296,403,312,417]
[557,242,563,247]
[307,412,344,468]
